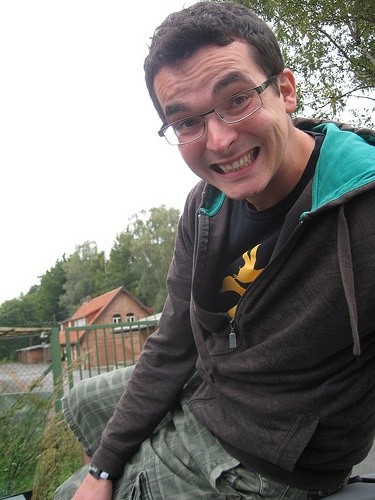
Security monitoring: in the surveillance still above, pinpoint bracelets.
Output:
[88,463,115,480]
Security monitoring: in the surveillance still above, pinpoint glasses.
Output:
[158,76,276,145]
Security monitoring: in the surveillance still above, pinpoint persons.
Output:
[54,2,375,500]
